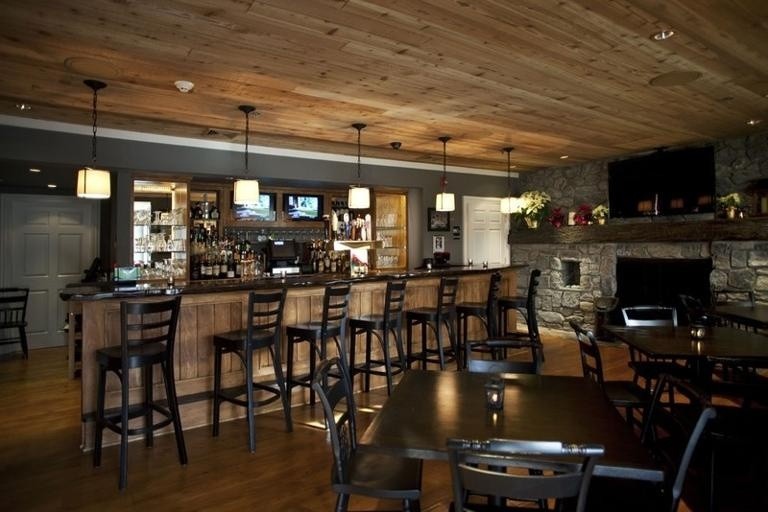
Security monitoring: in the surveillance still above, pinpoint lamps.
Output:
[77,79,111,200]
[500,147,521,214]
[348,123,370,209]
[435,137,456,212]
[233,105,260,205]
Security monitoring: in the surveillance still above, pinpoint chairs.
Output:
[348,279,407,397]
[554,368,717,511]
[445,436,606,512]
[212,286,293,453]
[0,287,30,360]
[311,357,423,511]
[464,339,543,376]
[456,273,503,371]
[498,269,545,366]
[93,295,188,490]
[569,320,652,442]
[621,305,678,409]
[679,294,722,328]
[286,284,351,416]
[406,277,459,371]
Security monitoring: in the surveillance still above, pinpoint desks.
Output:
[359,368,665,512]
[599,324,768,443]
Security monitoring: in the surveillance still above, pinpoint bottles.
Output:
[189,223,250,246]
[191,245,199,280]
[336,200,340,221]
[318,250,324,272]
[202,192,209,219]
[227,250,235,278]
[336,252,343,272]
[311,240,316,259]
[335,222,367,240]
[312,252,318,273]
[189,205,193,218]
[350,210,354,220]
[200,245,206,279]
[242,250,248,282]
[250,249,256,281]
[220,249,227,278]
[346,201,348,209]
[211,204,219,218]
[256,254,262,279]
[235,251,242,278]
[322,239,327,254]
[343,253,350,272]
[193,201,203,218]
[316,239,321,257]
[340,200,345,221]
[330,251,336,272]
[206,244,212,279]
[324,250,330,272]
[332,200,336,212]
[212,244,220,279]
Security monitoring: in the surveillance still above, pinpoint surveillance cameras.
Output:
[390,142,402,150]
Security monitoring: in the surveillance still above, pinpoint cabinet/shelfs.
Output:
[373,191,409,271]
[128,176,190,282]
[64,283,105,380]
[59,263,529,452]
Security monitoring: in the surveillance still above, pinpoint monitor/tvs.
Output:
[229,190,277,222]
[268,239,297,260]
[282,193,324,222]
[608,146,716,217]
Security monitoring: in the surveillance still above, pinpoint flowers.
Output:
[717,192,750,209]
[519,190,551,221]
[545,207,565,220]
[591,204,609,219]
[576,203,592,217]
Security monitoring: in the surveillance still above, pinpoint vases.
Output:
[597,217,606,225]
[521,209,545,229]
[580,215,588,226]
[724,206,737,219]
[552,221,563,228]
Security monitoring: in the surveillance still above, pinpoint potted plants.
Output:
[96,280,121,292]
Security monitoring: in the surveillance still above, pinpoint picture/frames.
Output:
[428,207,450,232]
[432,235,445,254]
